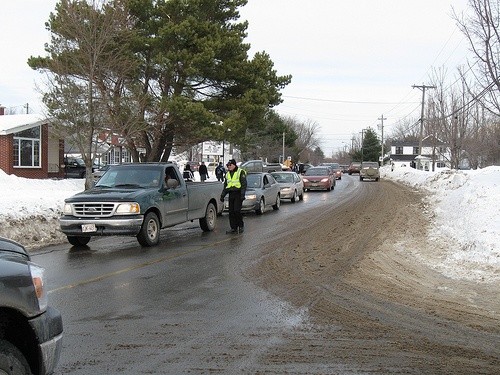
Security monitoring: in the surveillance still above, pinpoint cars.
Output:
[270,171,304,203]
[340,165,350,174]
[85,164,129,189]
[290,163,314,174]
[321,162,343,180]
[222,171,281,215]
[300,166,337,192]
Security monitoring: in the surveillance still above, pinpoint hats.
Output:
[226,159,236,165]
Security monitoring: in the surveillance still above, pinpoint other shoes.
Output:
[229,228,236,233]
[239,221,244,233]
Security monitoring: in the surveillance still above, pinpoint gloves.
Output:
[220,196,224,202]
[240,195,245,200]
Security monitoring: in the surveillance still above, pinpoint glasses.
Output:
[228,165,231,166]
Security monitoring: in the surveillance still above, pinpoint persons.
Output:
[220,159,248,235]
[290,161,303,174]
[187,162,226,182]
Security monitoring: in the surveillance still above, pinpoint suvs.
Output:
[359,161,381,182]
[349,162,362,175]
[0,236,64,375]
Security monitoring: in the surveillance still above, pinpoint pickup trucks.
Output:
[236,159,282,174]
[60,161,225,249]
